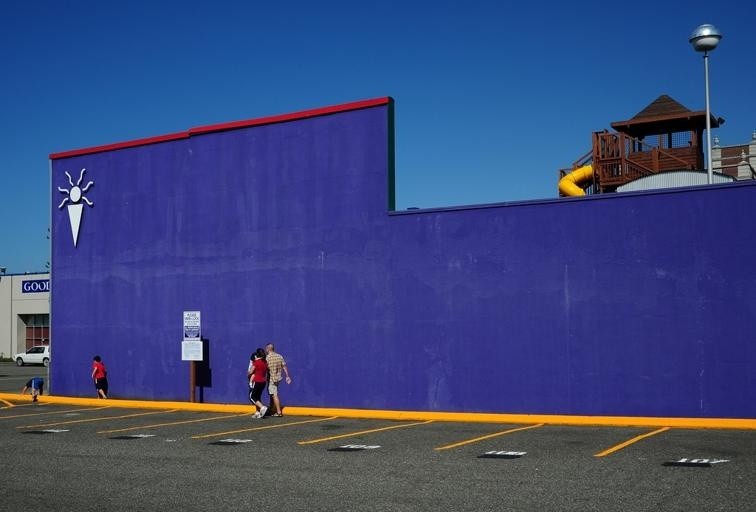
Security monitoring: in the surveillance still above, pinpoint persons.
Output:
[247,352,256,389]
[264,343,291,416]
[22,377,45,396]
[246,348,269,420]
[91,356,107,399]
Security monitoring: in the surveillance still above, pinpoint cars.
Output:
[15,345,50,367]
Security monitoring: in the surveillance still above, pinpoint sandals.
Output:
[270,413,283,417]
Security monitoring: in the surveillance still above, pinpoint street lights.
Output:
[689,23,723,184]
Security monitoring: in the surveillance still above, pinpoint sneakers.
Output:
[253,405,268,418]
